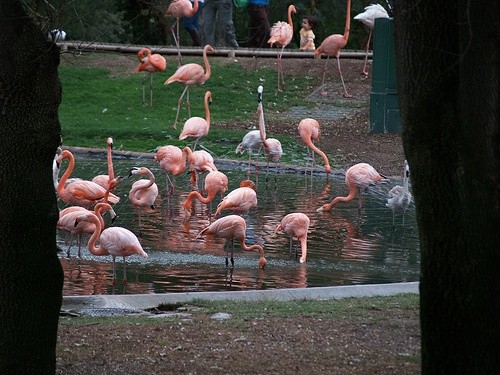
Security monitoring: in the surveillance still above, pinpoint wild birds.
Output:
[52,1,411,286]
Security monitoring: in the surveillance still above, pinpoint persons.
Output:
[185,0,317,52]
